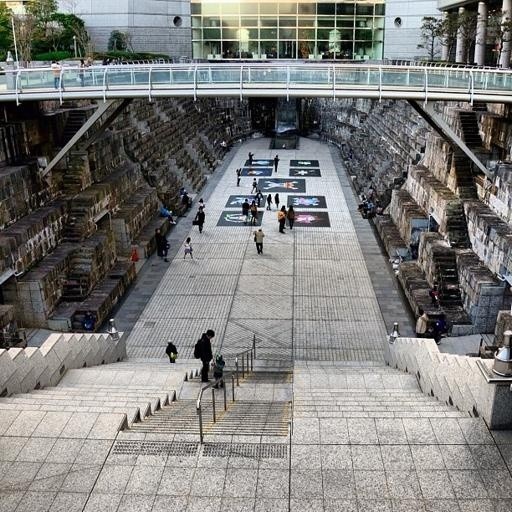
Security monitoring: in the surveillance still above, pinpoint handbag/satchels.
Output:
[171,351,177,359]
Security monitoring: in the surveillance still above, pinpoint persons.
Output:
[165,340,178,363]
[152,151,375,263]
[211,354,225,388]
[81,310,96,331]
[430,285,441,306]
[50,55,122,90]
[415,310,428,338]
[197,330,214,382]
[430,315,444,344]
